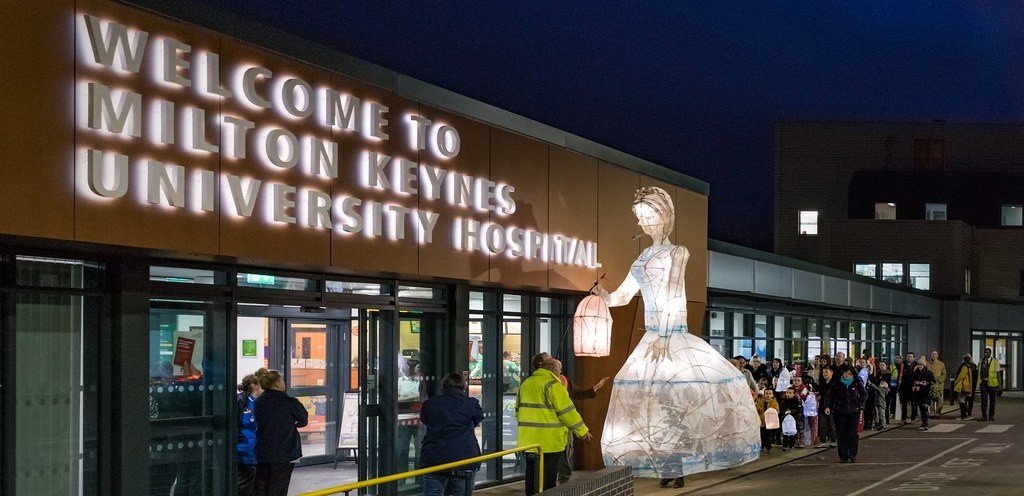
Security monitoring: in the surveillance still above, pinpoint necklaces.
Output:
[911,356,934,432]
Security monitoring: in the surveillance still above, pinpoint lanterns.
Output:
[572,294,613,357]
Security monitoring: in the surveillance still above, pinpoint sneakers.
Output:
[673,478,684,488]
[660,478,674,486]
[767,451,772,453]
[955,415,974,421]
[782,448,795,452]
[794,443,805,448]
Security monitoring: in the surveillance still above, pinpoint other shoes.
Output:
[814,442,829,448]
[918,425,929,430]
[828,442,838,447]
[977,416,987,421]
[989,418,996,421]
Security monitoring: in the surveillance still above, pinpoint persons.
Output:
[514,360,594,496]
[824,365,866,463]
[777,387,804,452]
[723,351,902,448]
[180,359,201,380]
[419,372,485,496]
[898,351,921,425]
[592,186,763,490]
[926,350,948,419]
[469,344,483,378]
[754,388,779,455]
[976,348,1005,422]
[951,355,979,422]
[236,368,265,496]
[253,368,309,495]
[398,358,426,488]
[502,350,520,390]
[530,352,610,487]
